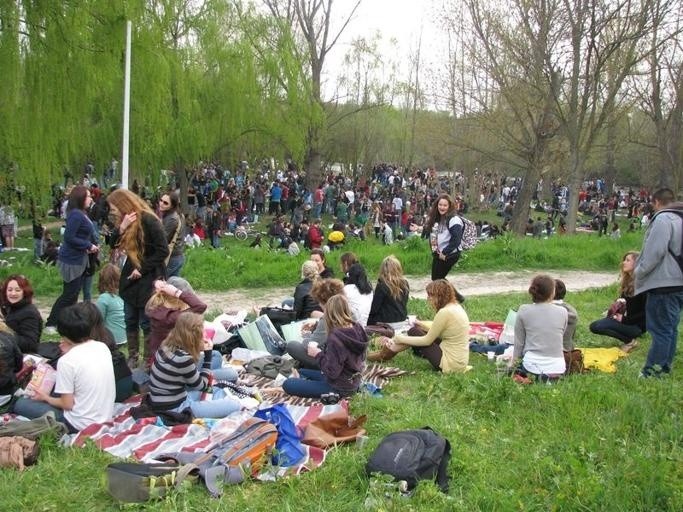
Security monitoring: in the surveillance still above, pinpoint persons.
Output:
[632,187,683,379]
[101,186,172,377]
[253,250,408,399]
[589,249,647,352]
[366,277,471,373]
[512,276,567,382]
[468,279,581,364]
[2,158,683,266]
[0,263,240,432]
[41,184,105,336]
[155,184,189,282]
[408,195,465,305]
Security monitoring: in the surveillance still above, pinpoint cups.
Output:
[487,352,494,360]
[407,314,415,327]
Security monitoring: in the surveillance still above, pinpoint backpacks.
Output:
[207,417,278,486]
[104,462,184,503]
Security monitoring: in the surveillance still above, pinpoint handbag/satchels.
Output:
[253,401,306,467]
[445,214,477,253]
[163,244,174,267]
[296,412,368,447]
[562,349,584,375]
[364,426,450,492]
[606,298,627,321]
[201,302,320,379]
[0,435,40,471]
[0,412,58,440]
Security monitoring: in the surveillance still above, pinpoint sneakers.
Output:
[40,326,60,337]
[620,338,641,353]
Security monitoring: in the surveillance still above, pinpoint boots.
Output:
[131,330,153,374]
[366,342,398,363]
[125,330,139,370]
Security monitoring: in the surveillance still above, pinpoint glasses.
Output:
[158,198,168,205]
